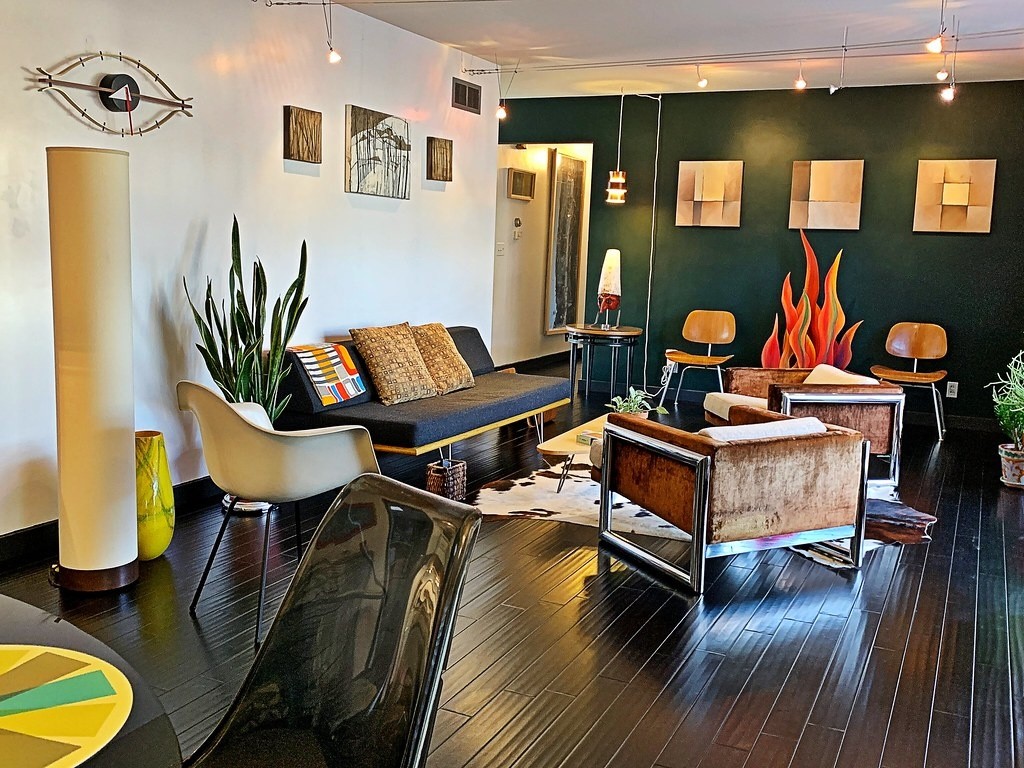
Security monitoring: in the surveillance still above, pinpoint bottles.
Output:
[135,429,176,562]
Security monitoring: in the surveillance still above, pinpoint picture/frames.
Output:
[507,168,537,200]
[541,149,587,335]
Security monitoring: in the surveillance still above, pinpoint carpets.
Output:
[474,456,937,570]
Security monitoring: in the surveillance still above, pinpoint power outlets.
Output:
[946,382,959,399]
[665,348,678,373]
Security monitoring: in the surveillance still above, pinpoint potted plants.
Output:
[605,387,669,421]
[984,350,1024,489]
[182,214,309,512]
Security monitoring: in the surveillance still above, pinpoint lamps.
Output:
[321,1,342,65]
[493,54,521,119]
[927,0,960,102]
[696,63,708,89]
[591,248,622,330]
[830,50,847,95]
[796,60,807,91]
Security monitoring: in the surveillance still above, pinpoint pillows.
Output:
[348,322,477,406]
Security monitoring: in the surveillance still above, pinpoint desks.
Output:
[566,324,642,404]
[0,594,183,767]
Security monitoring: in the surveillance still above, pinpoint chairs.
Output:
[598,366,906,593]
[182,472,484,768]
[176,379,384,646]
[658,310,735,410]
[870,322,948,441]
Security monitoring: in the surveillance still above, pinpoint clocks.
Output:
[606,95,627,204]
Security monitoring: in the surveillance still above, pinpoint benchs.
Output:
[266,327,572,462]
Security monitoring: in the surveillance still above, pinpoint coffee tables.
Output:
[536,404,650,493]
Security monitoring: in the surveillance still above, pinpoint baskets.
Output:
[426,459,467,502]
